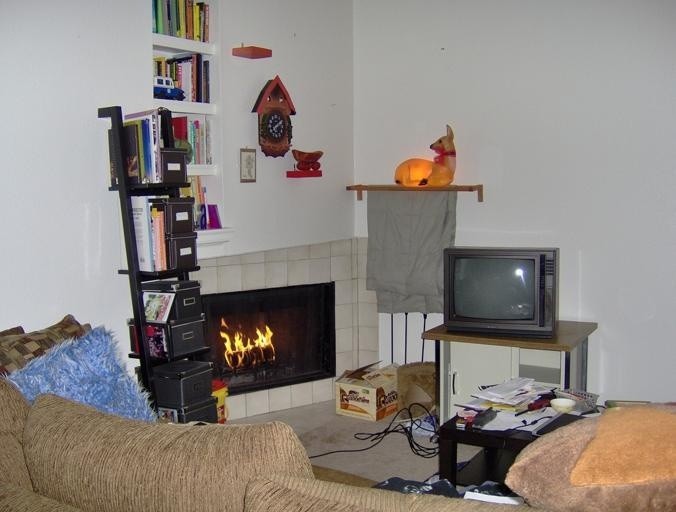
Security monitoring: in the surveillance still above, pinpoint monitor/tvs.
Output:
[441,245,562,342]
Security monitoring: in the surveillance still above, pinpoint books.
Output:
[172,115,213,165]
[113,194,169,273]
[150,0,211,42]
[457,374,574,433]
[153,52,211,102]
[179,177,221,231]
[107,108,175,185]
[129,325,168,357]
[142,291,177,324]
[464,480,524,505]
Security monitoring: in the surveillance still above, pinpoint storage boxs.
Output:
[139,279,204,319]
[159,148,188,183]
[152,398,218,426]
[164,233,198,271]
[152,202,194,238]
[335,357,398,425]
[152,358,214,405]
[128,316,207,355]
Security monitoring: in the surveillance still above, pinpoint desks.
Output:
[422,319,598,425]
[434,387,597,488]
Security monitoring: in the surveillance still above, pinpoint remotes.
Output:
[471,409,497,427]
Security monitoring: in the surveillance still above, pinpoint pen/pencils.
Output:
[515,408,529,416]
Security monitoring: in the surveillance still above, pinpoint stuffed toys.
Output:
[395,123,457,187]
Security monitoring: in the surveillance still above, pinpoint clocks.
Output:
[252,74,297,158]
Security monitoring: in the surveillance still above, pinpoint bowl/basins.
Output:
[550,398,576,414]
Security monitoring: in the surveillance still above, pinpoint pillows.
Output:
[503,402,676,509]
[5,326,156,425]
[0,311,91,375]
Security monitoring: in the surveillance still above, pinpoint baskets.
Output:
[554,388,599,413]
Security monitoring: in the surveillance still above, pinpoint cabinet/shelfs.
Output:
[98,105,221,426]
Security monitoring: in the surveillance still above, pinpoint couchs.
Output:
[0,312,525,510]
